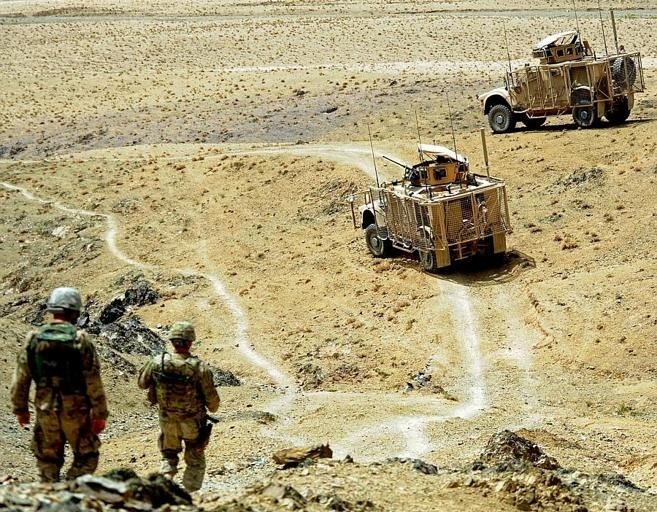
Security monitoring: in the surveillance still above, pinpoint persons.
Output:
[137,321,220,492]
[10,287,109,483]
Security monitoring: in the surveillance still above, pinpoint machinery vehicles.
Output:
[475,0,646,134]
[346,88,517,275]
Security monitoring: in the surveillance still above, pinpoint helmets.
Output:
[45,286,81,314]
[167,322,196,342]
